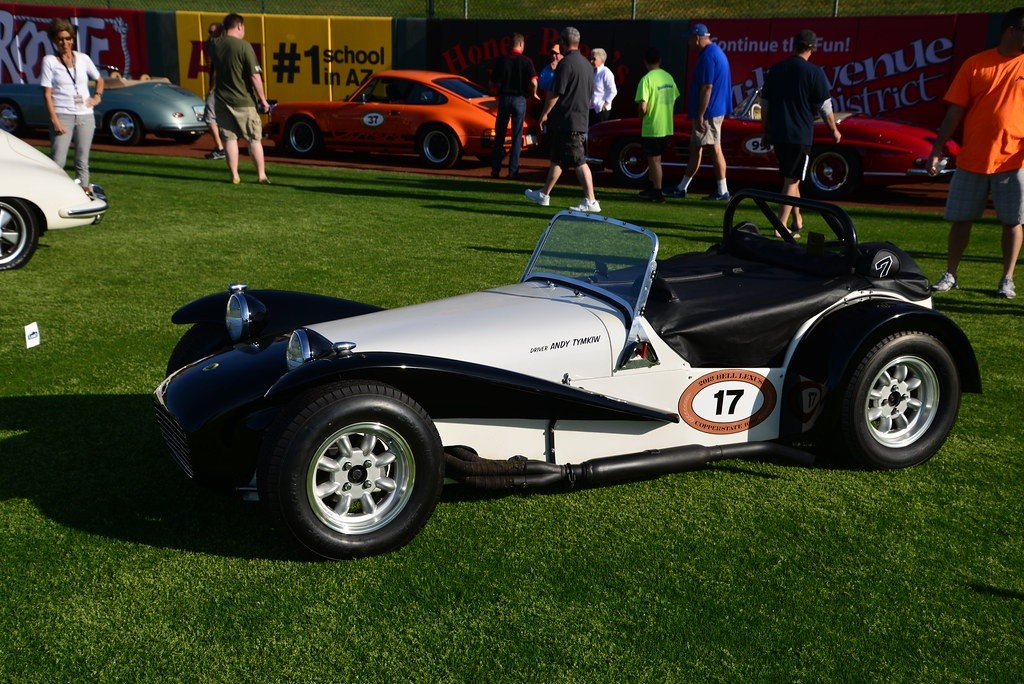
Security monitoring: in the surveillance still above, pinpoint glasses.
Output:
[57,36,73,42]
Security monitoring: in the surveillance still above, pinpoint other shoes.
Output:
[701,191,730,201]
[504,172,519,180]
[639,188,661,197]
[666,188,686,197]
[258,177,272,184]
[231,176,241,184]
[490,170,500,177]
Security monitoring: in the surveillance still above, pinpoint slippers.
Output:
[774,229,798,239]
[790,221,803,231]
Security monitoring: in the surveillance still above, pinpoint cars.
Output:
[0,63,212,146]
[0,130,108,273]
[584,88,939,201]
[265,70,542,171]
[152,189,982,562]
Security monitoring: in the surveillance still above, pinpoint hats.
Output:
[551,44,560,54]
[794,29,816,47]
[686,24,710,37]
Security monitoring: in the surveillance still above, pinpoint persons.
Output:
[39,19,104,196]
[588,48,617,127]
[761,29,841,239]
[489,32,540,180]
[632,46,680,205]
[202,13,273,184]
[926,6,1024,300]
[662,23,733,200]
[539,44,564,100]
[524,26,602,213]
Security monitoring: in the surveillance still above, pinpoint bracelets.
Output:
[94,92,102,95]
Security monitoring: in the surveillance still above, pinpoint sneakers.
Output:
[570,197,602,212]
[997,277,1017,298]
[204,148,226,159]
[930,272,959,290]
[525,188,550,206]
